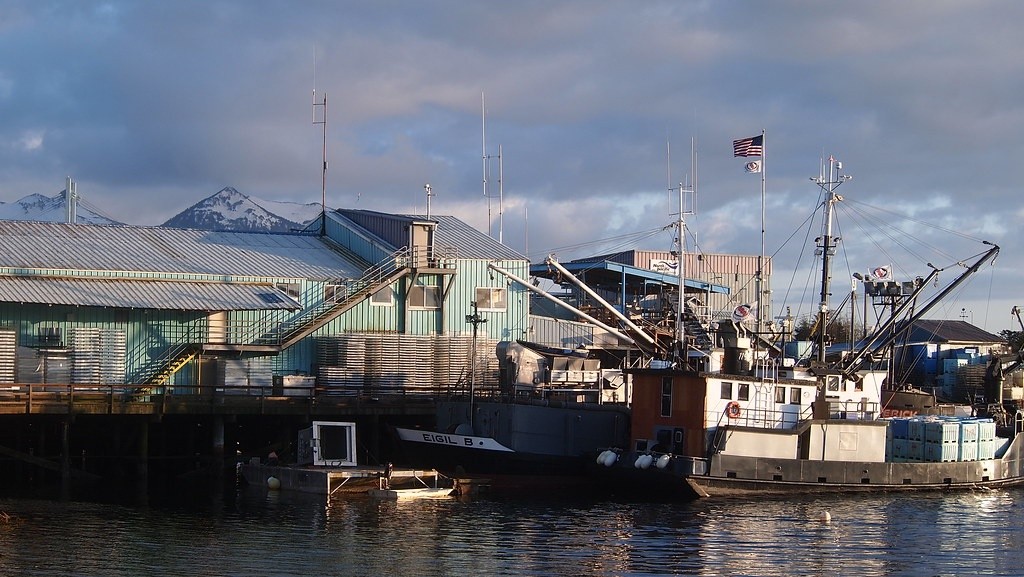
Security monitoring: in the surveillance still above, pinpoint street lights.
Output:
[862,281,918,392]
[463,301,490,426]
[423,182,433,219]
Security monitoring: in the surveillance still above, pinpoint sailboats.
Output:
[488,129,1024,503]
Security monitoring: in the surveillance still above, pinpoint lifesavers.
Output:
[726,401,741,419]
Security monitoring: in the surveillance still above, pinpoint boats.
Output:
[369,470,460,502]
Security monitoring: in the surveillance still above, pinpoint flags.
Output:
[733,134,763,158]
[731,300,758,322]
[868,263,893,280]
[744,160,761,174]
